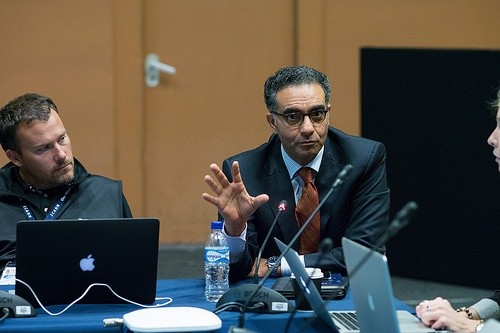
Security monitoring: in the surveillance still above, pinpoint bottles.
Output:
[204,221,229,302]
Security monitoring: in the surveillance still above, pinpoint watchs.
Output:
[267,256,281,277]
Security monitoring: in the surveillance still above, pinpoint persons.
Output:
[0,93,133,278]
[416,89,500,333]
[201,66,390,278]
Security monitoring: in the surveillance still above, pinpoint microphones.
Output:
[231,164,354,333]
[297,202,420,333]
[283,236,334,333]
[253,200,288,285]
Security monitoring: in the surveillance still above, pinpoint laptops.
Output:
[341,237,436,333]
[274,236,361,333]
[15,217,162,307]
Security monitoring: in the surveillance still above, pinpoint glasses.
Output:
[270,105,329,127]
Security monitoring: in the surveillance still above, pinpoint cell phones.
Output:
[323,271,331,280]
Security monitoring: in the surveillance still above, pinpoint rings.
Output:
[426,305,430,311]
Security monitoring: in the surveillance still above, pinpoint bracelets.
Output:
[473,320,485,333]
[456,307,473,319]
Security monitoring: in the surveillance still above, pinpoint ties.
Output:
[296,167,321,255]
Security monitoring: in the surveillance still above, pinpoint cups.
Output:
[291,267,324,312]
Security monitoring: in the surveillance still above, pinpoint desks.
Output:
[0,276,453,333]
